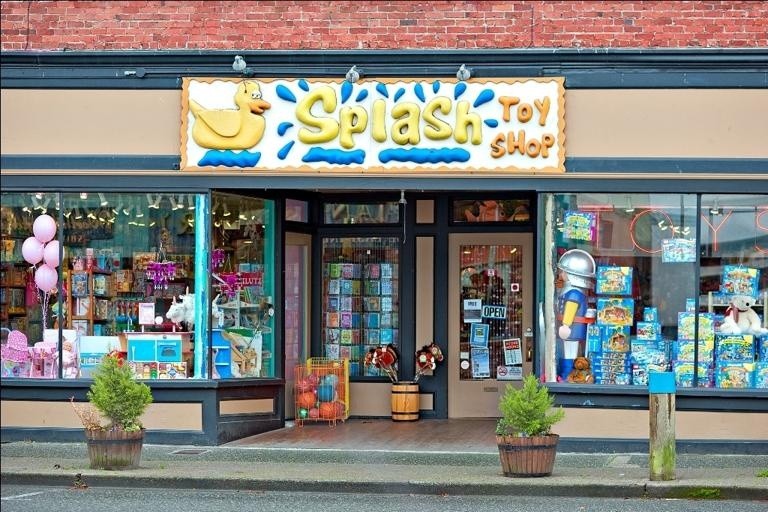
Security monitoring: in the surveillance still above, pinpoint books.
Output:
[323,263,398,375]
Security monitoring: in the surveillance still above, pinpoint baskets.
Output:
[294,364,345,428]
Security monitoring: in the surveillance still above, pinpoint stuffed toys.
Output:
[364,343,443,384]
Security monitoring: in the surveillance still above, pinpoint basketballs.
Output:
[297,374,338,419]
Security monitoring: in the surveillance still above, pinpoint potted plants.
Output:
[485,368,563,479]
[71,354,151,469]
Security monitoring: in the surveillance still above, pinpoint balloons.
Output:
[33,215,56,242]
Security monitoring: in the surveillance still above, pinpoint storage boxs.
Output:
[125,334,185,362]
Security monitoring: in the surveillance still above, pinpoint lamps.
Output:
[394,186,408,208]
[710,198,721,216]
[346,64,364,83]
[229,53,254,77]
[621,195,636,213]
[15,193,261,229]
[455,63,475,80]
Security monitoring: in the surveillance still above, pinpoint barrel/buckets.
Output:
[84,427,146,471]
[496,431,560,475]
[390,381,420,421]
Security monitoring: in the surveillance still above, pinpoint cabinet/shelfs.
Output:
[122,328,194,379]
[217,287,262,330]
[224,333,258,375]
[66,269,150,339]
[198,329,231,377]
[0,230,29,343]
